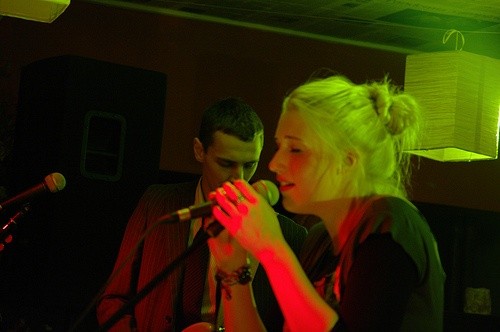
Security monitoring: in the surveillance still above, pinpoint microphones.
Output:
[160,179,280,225]
[0,172,67,209]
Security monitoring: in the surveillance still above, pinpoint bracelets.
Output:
[221,265,255,287]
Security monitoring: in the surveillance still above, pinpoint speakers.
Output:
[15,54,166,312]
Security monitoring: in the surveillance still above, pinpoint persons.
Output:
[204,75,445,332]
[97,97,308,332]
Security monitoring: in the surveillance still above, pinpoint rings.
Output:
[233,192,246,205]
